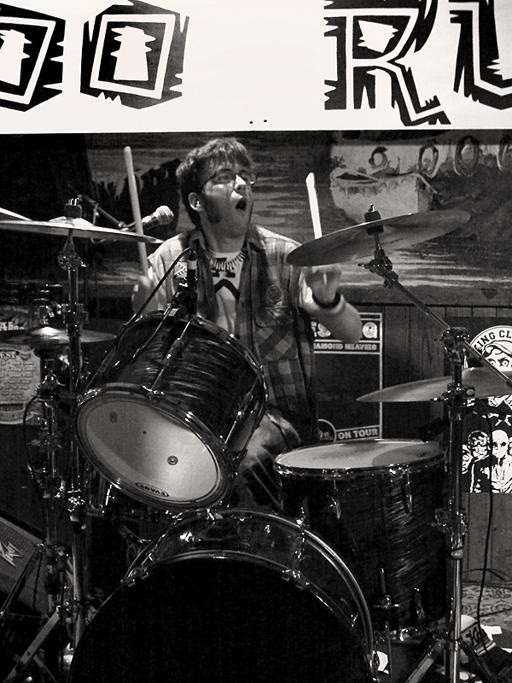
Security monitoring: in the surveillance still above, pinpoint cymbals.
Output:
[357,367,511,403]
[287,210,470,265]
[0,325,116,344]
[0,217,165,246]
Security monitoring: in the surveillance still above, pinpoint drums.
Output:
[71,509,372,678]
[76,307,269,512]
[271,440,452,645]
[1,516,75,678]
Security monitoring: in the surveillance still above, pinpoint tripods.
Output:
[0,255,512,682]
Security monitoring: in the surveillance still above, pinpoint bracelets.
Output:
[311,292,346,316]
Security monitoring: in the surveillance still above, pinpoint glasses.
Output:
[197,166,257,195]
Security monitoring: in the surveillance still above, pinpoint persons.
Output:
[129,135,364,521]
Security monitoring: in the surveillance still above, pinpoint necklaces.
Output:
[205,247,247,271]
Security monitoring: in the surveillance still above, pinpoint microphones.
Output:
[99,205,175,246]
